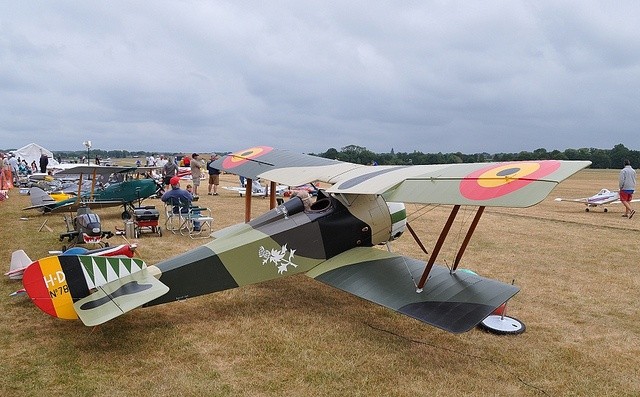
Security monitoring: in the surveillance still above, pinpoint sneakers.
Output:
[213,192,218,194]
[628,209,635,218]
[208,192,212,195]
[621,213,629,217]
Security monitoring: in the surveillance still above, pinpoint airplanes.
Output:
[57,205,115,253]
[221,179,287,196]
[555,188,638,213]
[20,162,165,220]
[21,172,119,200]
[25,144,594,337]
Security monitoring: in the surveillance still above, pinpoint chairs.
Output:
[164,196,201,236]
[177,197,213,240]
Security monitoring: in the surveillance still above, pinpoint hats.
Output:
[170,175,181,184]
[192,153,199,157]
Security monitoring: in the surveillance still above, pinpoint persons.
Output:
[200,157,207,167]
[0,143,53,191]
[240,175,260,188]
[190,153,201,197]
[58,154,112,167]
[207,153,220,196]
[136,154,190,191]
[160,176,206,231]
[619,159,636,219]
